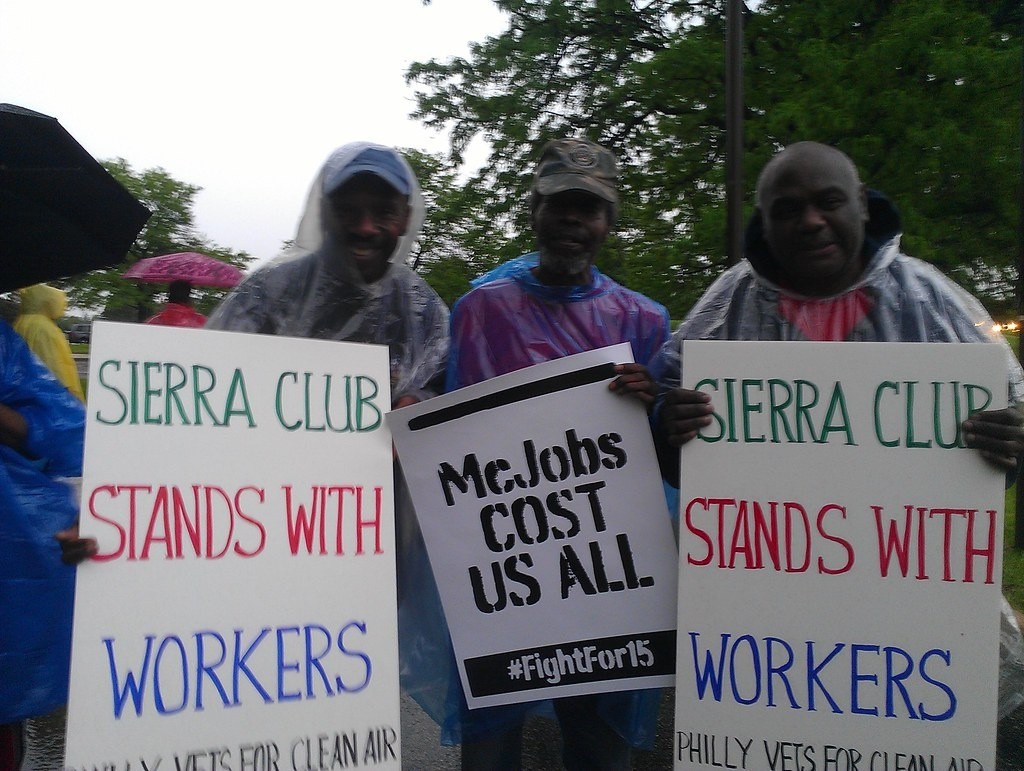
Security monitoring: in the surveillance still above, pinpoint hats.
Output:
[530,137,620,204]
[319,141,415,199]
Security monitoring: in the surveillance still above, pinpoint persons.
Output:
[0,315,99,771]
[11,284,87,405]
[144,280,210,330]
[449,139,671,771]
[648,139,1024,490]
[202,146,451,457]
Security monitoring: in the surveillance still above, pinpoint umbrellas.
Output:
[0,101,155,299]
[120,250,249,288]
